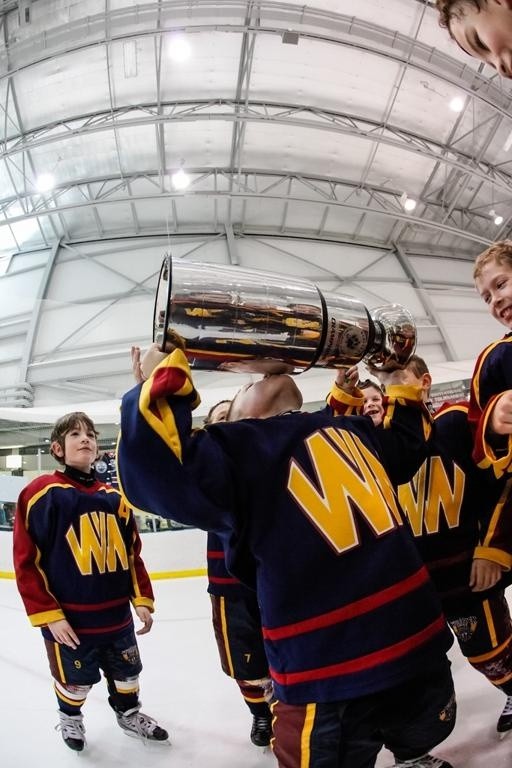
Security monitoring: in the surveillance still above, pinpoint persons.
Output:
[324,364,385,429]
[115,346,456,767]
[92,451,119,489]
[203,401,272,746]
[13,412,168,751]
[467,241,511,481]
[133,512,169,533]
[0,502,15,530]
[379,356,512,730]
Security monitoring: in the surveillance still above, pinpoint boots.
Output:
[108,696,168,741]
[57,707,84,752]
[394,753,452,768]
[495,695,512,732]
[246,699,273,747]
